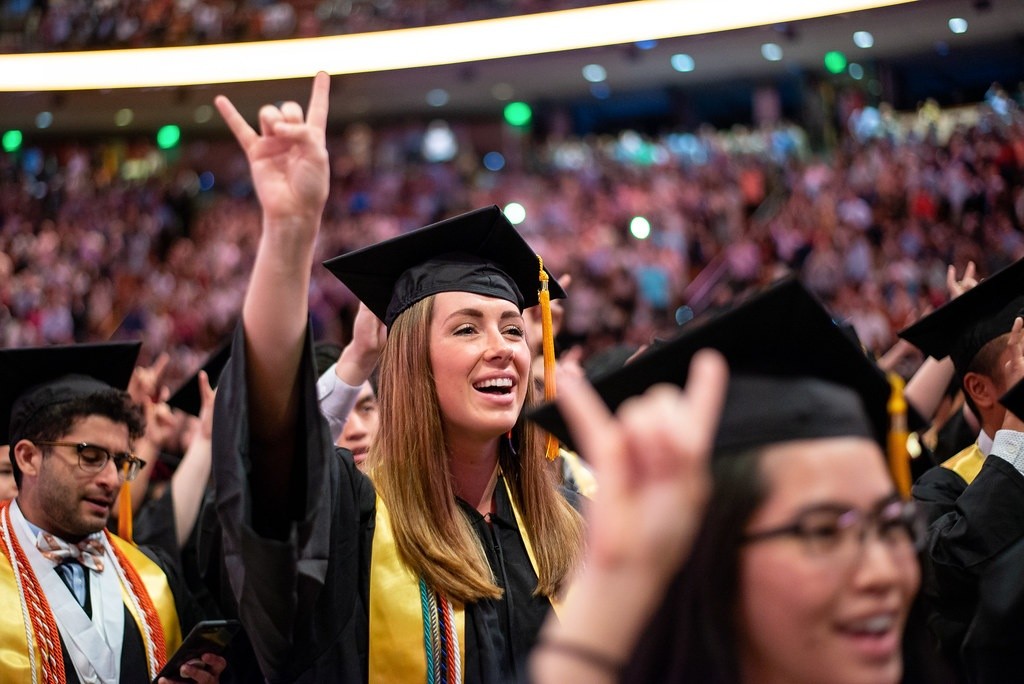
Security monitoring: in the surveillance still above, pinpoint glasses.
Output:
[732,501,929,559]
[44,439,147,481]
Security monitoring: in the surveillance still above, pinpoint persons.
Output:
[0,345,239,684]
[0,1,1024,683]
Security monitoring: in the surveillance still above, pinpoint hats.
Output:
[997,376,1024,422]
[526,272,931,457]
[165,337,234,417]
[322,205,568,337]
[0,340,143,542]
[897,256,1024,361]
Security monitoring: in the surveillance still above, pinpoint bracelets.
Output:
[533,630,625,675]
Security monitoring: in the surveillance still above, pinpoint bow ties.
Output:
[36,534,105,572]
